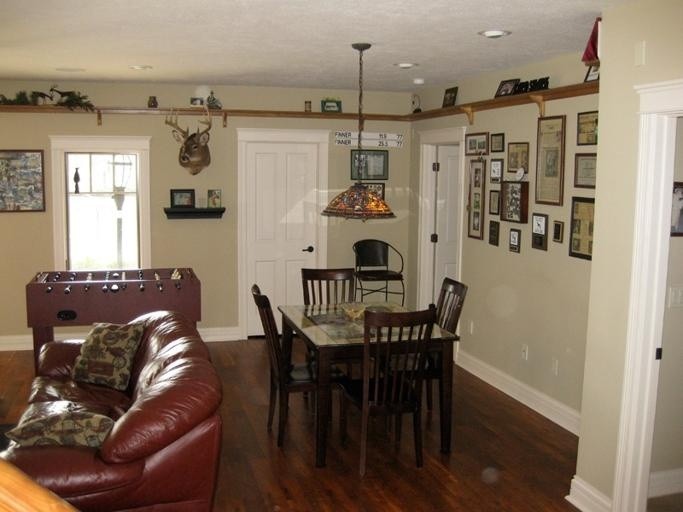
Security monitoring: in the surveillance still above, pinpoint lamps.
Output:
[323,44,396,219]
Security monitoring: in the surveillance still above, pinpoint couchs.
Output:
[5,308,222,512]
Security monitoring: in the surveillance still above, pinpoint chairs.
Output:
[251,284,347,447]
[352,239,405,306]
[339,304,436,478]
[389,277,468,410]
[300,269,357,402]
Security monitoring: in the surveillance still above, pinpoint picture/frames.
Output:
[583,64,599,82]
[670,181,683,236]
[442,87,459,107]
[465,110,599,261]
[361,183,385,201]
[351,149,389,180]
[494,78,519,97]
[0,150,46,212]
[170,189,195,208]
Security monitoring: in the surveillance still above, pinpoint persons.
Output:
[210,191,218,208]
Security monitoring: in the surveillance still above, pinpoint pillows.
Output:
[71,321,144,389]
[4,412,113,447]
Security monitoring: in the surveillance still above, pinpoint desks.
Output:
[278,304,460,467]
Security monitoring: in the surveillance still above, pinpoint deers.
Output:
[163,102,214,176]
